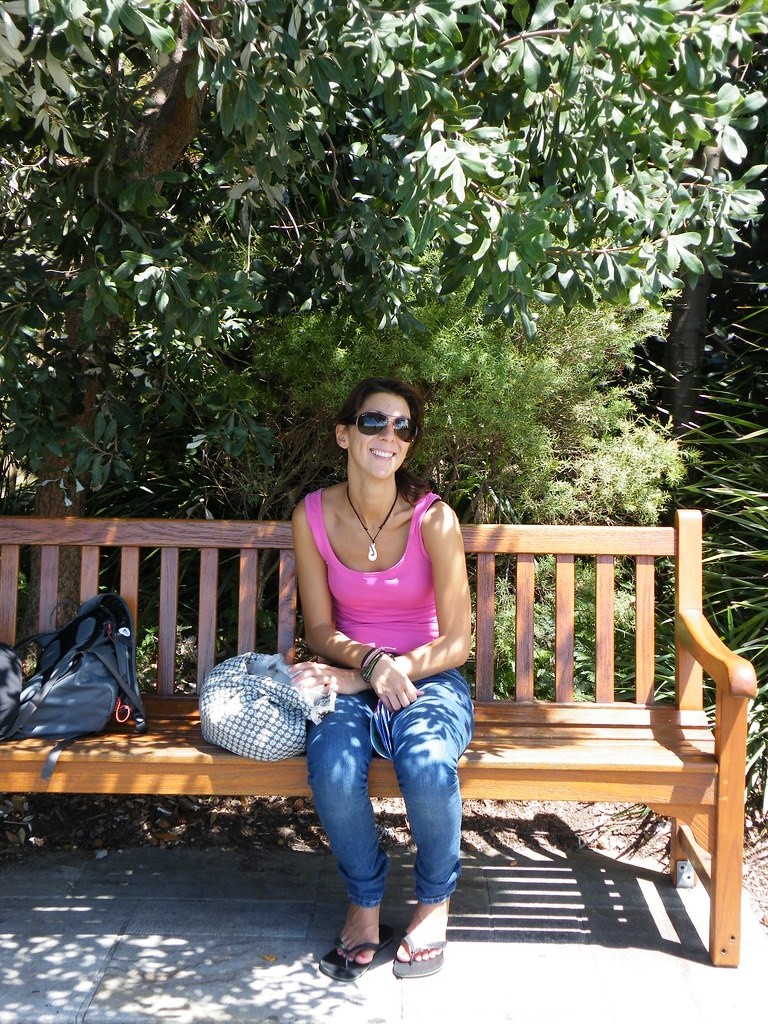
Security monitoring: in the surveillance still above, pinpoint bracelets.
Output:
[360,646,396,683]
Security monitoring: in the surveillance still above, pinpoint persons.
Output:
[286,376,472,982]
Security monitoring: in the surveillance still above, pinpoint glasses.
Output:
[341,411,421,445]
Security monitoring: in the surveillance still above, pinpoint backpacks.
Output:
[19,592,150,739]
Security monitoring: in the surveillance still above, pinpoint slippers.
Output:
[319,924,450,981]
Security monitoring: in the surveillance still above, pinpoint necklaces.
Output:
[347,485,398,561]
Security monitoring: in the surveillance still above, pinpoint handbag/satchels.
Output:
[201,652,336,761]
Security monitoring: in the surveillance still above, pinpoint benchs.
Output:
[1,509,757,967]
